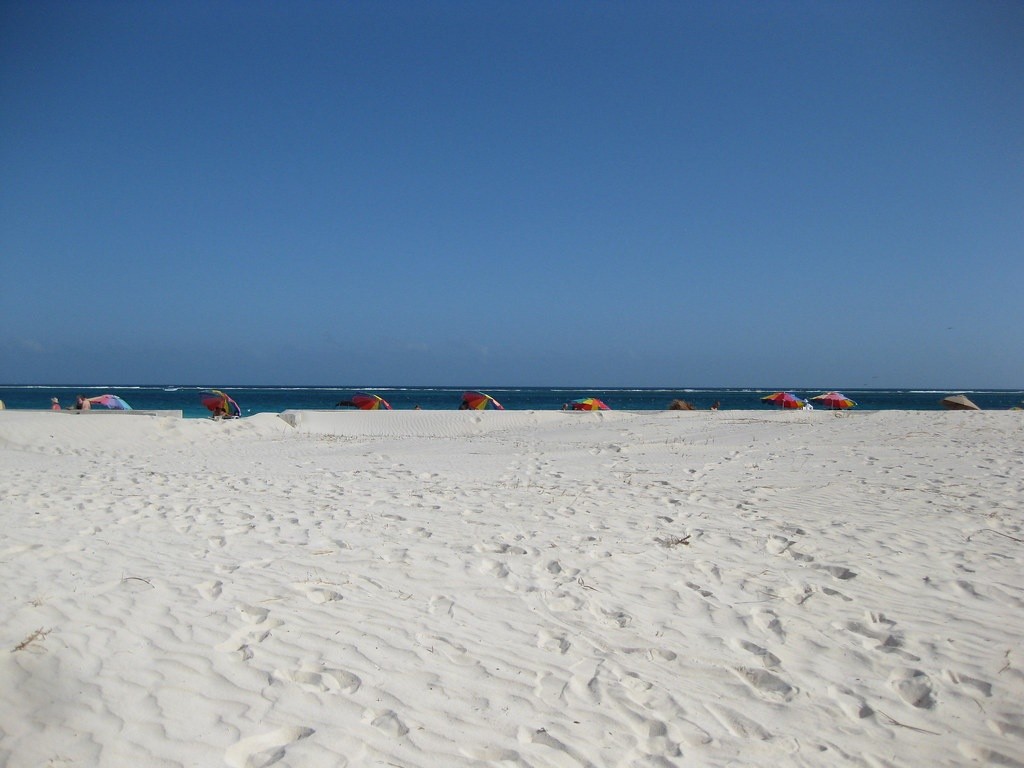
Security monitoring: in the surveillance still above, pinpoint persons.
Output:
[415,405,422,410]
[50,396,61,410]
[212,407,232,418]
[710,402,720,410]
[75,393,91,410]
[562,403,568,411]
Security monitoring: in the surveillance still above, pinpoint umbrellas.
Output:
[463,391,505,410]
[809,391,858,410]
[198,389,241,420]
[570,397,611,410]
[88,394,134,410]
[760,391,807,410]
[943,394,980,410]
[1009,406,1024,410]
[338,399,357,409]
[352,391,392,410]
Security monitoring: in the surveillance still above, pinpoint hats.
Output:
[802,399,809,402]
[51,397,59,403]
